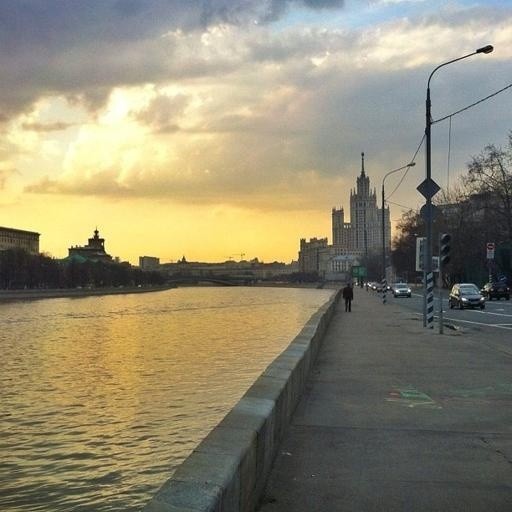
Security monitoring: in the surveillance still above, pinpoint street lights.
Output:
[380,161,417,305]
[420,43,495,328]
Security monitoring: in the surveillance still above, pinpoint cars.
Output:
[355,280,413,300]
[447,281,510,310]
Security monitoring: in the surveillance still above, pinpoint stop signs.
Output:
[486,243,494,259]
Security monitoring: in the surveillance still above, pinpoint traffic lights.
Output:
[438,231,451,267]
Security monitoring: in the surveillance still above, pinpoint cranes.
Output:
[232,251,248,261]
[224,255,233,260]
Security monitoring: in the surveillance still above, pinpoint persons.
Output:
[343,283,353,313]
